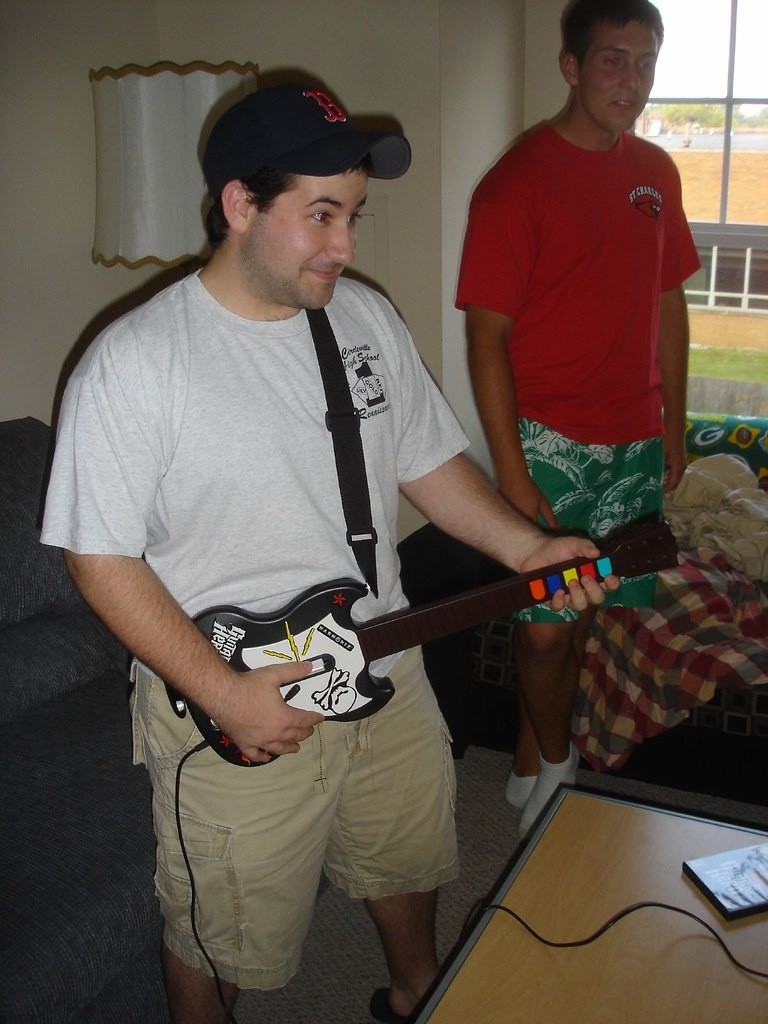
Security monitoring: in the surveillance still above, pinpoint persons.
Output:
[455,0,705,840]
[37,82,621,1024]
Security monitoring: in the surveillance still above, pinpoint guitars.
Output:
[182,520,680,768]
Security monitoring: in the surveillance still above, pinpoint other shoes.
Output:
[370,987,409,1024]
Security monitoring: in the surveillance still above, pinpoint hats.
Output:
[203,83,411,179]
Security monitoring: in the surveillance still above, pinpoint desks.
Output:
[406,782,768,1024]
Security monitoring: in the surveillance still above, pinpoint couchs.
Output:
[0,421,471,1024]
[400,420,768,805]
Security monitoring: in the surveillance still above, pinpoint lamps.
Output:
[86,58,255,268]
